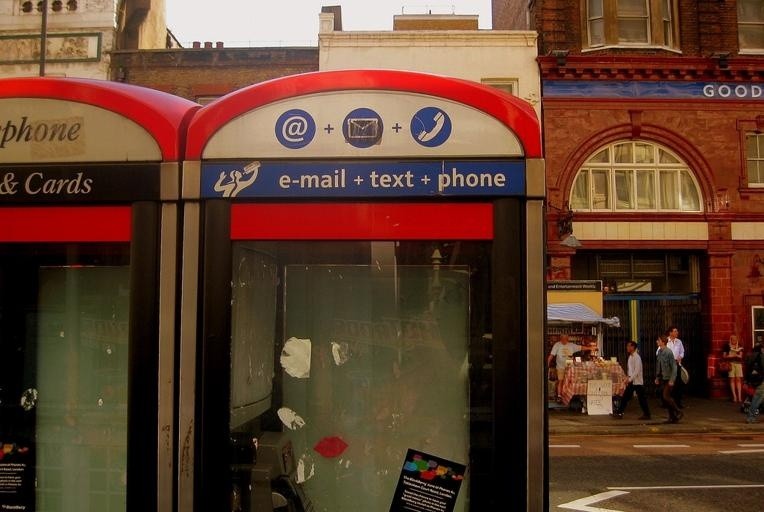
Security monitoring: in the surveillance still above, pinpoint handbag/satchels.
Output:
[718,362,732,373]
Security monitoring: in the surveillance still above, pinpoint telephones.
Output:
[248,435,314,512]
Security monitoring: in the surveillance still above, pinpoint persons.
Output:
[720,333,745,404]
[654,325,691,412]
[744,341,763,426]
[651,334,684,424]
[609,341,652,422]
[547,334,600,402]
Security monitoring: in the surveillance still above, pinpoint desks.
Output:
[564,365,622,415]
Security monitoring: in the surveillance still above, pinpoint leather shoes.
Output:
[638,415,651,420]
[609,412,625,418]
[663,411,683,424]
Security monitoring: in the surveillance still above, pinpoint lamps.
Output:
[558,211,582,248]
